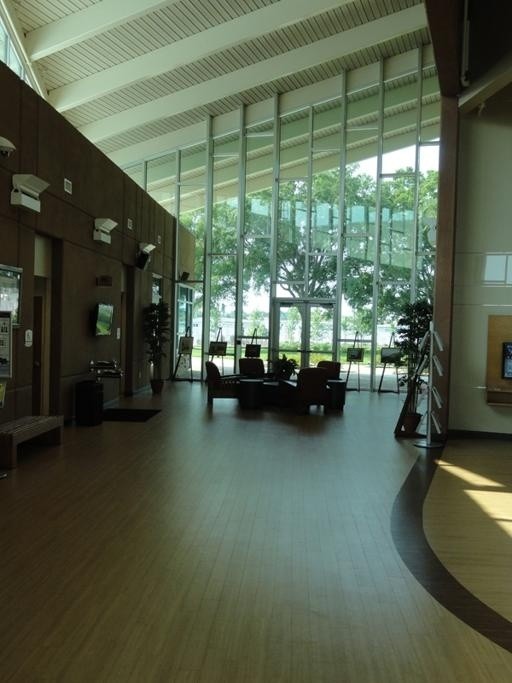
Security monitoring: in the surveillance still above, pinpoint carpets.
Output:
[101,408,161,423]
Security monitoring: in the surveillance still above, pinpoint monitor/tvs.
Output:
[0,264,24,332]
[501,341,512,379]
[95,303,114,338]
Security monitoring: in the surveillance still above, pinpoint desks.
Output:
[323,379,347,415]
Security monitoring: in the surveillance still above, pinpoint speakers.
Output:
[134,251,150,271]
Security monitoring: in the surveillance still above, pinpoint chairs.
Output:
[205,360,248,406]
[317,361,343,380]
[239,358,266,379]
[285,368,324,415]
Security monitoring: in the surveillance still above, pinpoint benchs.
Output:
[1,415,64,468]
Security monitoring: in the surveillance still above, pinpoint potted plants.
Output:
[143,298,170,396]
[389,295,433,440]
[267,354,298,381]
